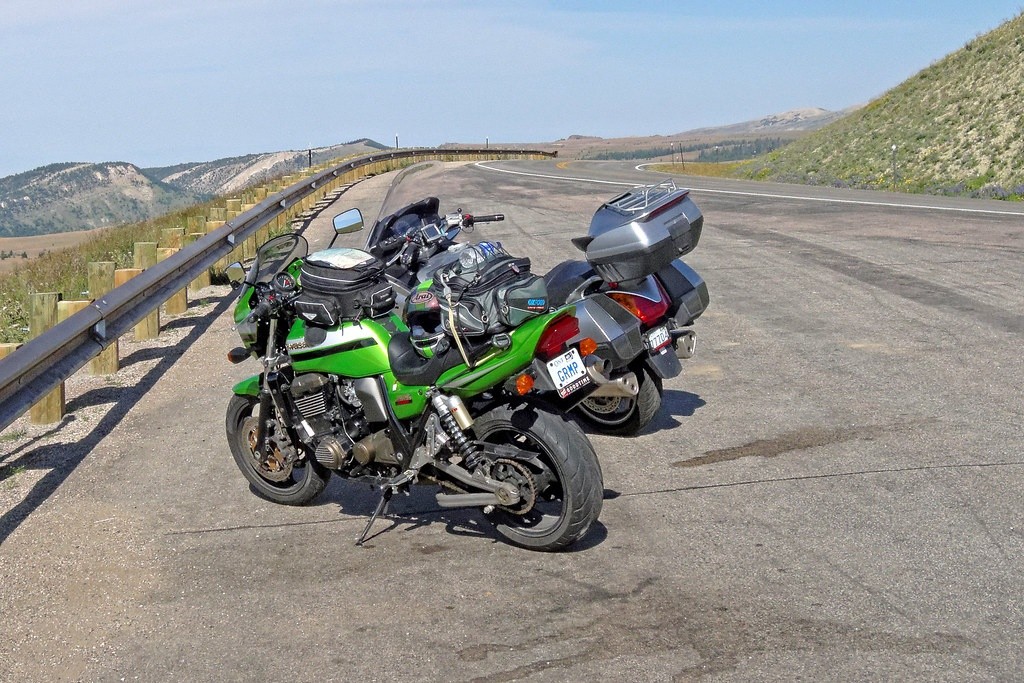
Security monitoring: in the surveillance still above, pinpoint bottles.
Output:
[459,241,501,269]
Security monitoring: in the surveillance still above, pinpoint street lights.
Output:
[892,146,897,189]
[670,143,675,165]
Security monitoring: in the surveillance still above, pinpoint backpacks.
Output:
[412,240,550,343]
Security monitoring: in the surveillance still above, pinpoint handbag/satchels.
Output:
[294,245,400,327]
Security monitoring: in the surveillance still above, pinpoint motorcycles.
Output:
[357,160,712,437]
[206,208,612,551]
